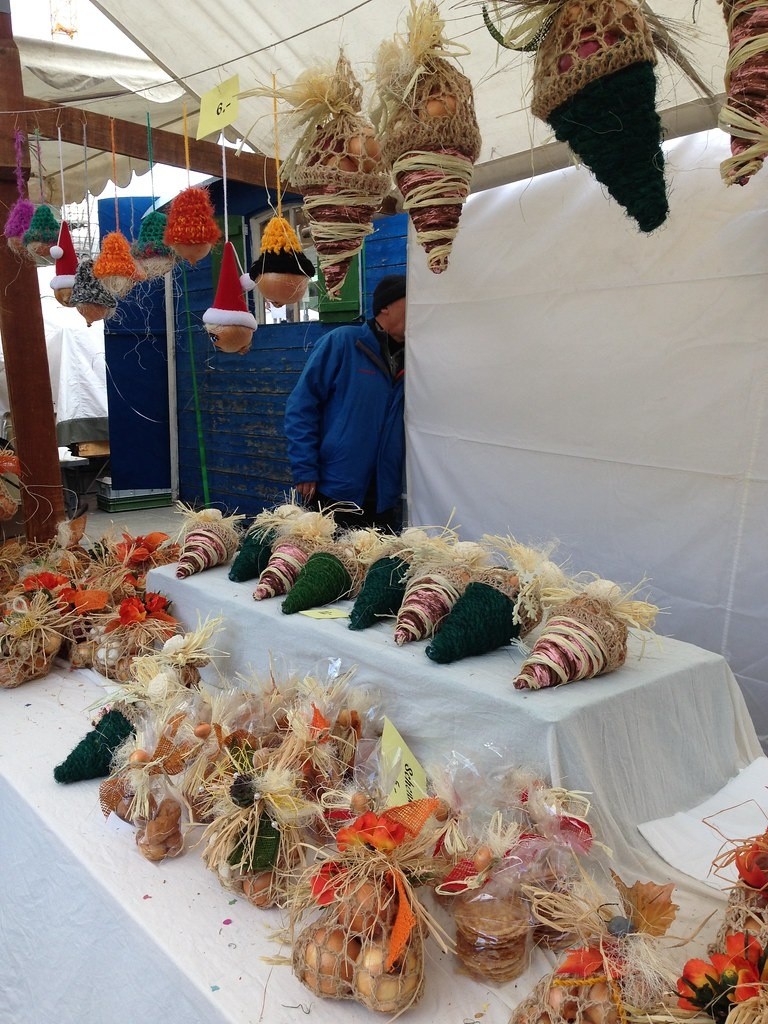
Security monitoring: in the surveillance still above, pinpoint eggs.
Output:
[0,0,766,1023]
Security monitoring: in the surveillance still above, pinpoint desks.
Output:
[144,552,725,849]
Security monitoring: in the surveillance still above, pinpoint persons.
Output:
[284,274,406,542]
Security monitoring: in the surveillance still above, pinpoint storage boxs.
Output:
[94,477,176,515]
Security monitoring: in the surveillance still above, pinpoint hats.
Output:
[373,274,406,316]
